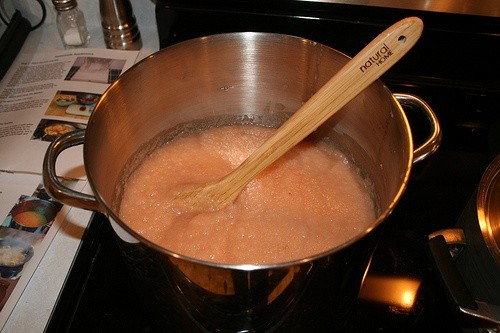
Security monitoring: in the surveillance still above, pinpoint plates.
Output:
[66,104,94,116]
[44,124,76,136]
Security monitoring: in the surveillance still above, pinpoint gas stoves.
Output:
[42,76,500,333]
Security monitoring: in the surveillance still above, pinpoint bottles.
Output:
[50,0,91,49]
[99,0,143,50]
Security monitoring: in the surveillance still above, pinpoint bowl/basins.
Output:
[0,240,34,278]
[76,94,99,105]
[53,96,76,106]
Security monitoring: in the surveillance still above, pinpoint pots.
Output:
[11,199,58,232]
[427,155,500,324]
[42,31,443,273]
